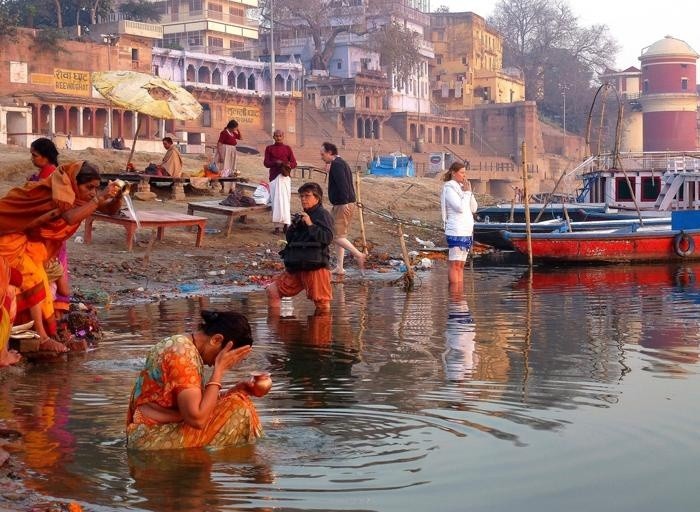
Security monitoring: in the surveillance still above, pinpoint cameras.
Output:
[292,212,305,227]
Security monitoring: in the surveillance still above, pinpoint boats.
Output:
[474,149,700,265]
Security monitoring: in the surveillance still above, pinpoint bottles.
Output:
[250,371,273,398]
[113,178,131,196]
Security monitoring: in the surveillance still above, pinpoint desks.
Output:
[185,200,272,236]
[84,209,209,253]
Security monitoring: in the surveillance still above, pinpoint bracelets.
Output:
[204,381,224,390]
[91,195,101,206]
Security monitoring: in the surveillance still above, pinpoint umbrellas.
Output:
[92,69,205,173]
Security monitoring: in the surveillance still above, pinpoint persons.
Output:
[262,128,298,237]
[29,137,72,303]
[214,119,242,194]
[264,181,336,322]
[439,161,479,286]
[441,283,477,402]
[319,142,368,276]
[112,137,123,150]
[0,369,90,504]
[1,159,131,369]
[143,137,184,177]
[335,282,370,408]
[126,453,273,511]
[65,134,72,149]
[104,122,110,149]
[122,309,273,453]
[265,316,332,424]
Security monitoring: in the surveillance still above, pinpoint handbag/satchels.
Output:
[283,240,323,267]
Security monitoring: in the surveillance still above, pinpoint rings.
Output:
[115,192,117,194]
